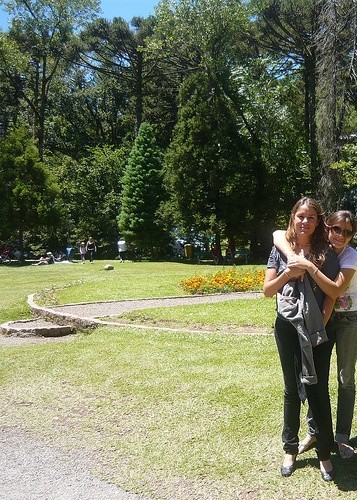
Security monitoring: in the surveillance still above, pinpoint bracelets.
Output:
[284,271,290,278]
[313,268,318,274]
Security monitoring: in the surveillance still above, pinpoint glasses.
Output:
[332,226,355,237]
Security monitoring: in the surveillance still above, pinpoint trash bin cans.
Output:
[184,244,192,257]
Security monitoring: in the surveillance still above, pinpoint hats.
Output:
[81,242,85,244]
[47,251,52,255]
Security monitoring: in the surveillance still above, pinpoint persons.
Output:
[226,246,233,260]
[117,237,126,262]
[80,242,86,264]
[262,197,341,481]
[211,244,220,264]
[38,251,55,265]
[272,209,357,459]
[85,237,97,263]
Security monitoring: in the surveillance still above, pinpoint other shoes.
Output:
[83,262,85,264]
[320,460,335,481]
[297,434,317,454]
[281,450,297,477]
[337,440,354,459]
